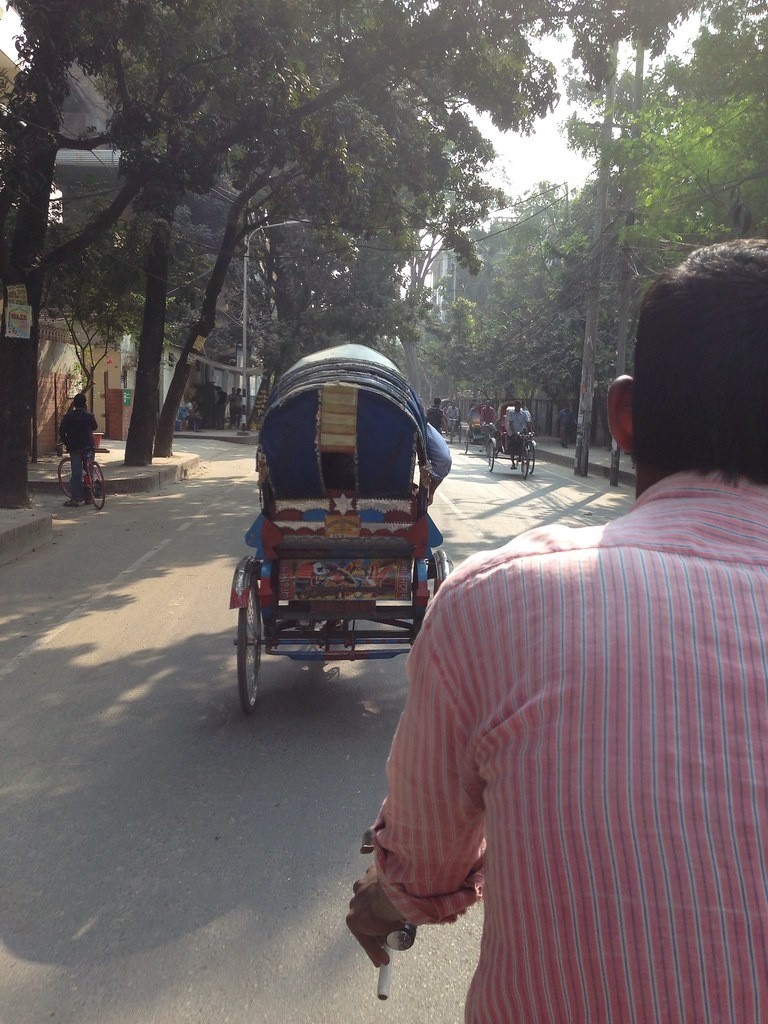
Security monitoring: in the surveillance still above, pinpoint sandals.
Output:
[86,500,94,504]
[63,500,79,507]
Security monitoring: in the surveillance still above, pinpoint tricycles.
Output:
[436,396,537,479]
[228,342,454,714]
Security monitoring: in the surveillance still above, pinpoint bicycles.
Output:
[57,446,106,511]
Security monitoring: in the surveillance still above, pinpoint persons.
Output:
[185,381,242,432]
[416,413,452,505]
[555,404,575,449]
[58,393,99,505]
[426,394,535,471]
[344,236,767,1024]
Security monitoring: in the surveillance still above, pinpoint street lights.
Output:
[237,218,314,423]
